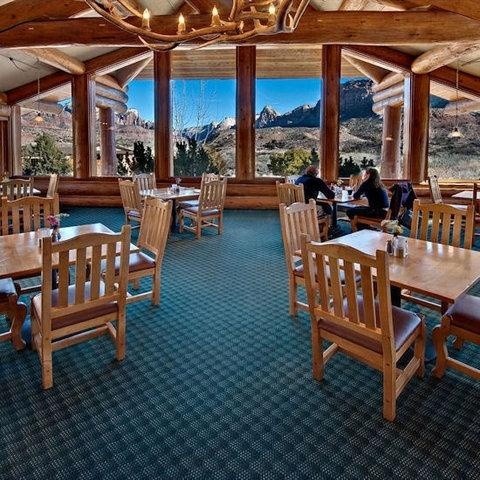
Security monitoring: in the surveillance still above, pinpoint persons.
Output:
[295,164,335,239]
[346,168,390,231]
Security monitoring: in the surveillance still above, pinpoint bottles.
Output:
[52,232,59,242]
[393,238,405,257]
[384,239,392,256]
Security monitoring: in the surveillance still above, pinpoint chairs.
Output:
[29,224,131,389]
[349,175,363,196]
[299,234,428,421]
[352,183,417,233]
[430,295,480,380]
[132,172,156,190]
[118,178,143,230]
[88,196,173,321]
[276,180,305,205]
[1,192,59,299]
[428,175,474,227]
[2,177,34,203]
[401,199,476,316]
[179,178,226,240]
[280,198,362,316]
[177,172,220,226]
[471,183,480,223]
[0,278,28,351]
[47,173,59,200]
